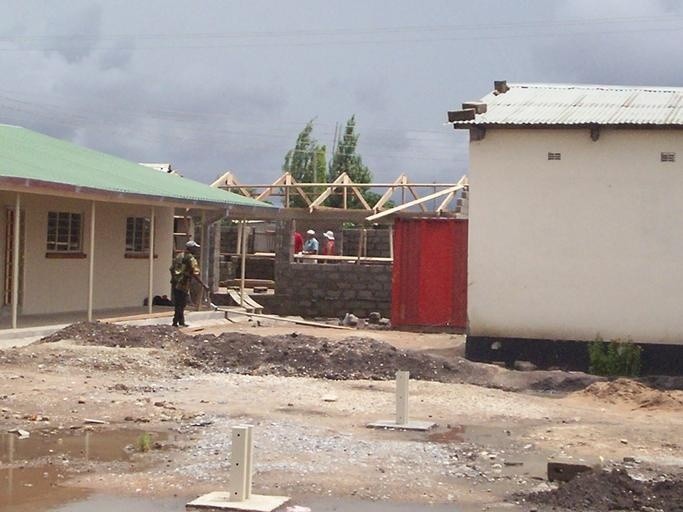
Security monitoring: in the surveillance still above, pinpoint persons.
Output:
[320,230,337,267]
[293,229,303,265]
[168,238,210,329]
[298,228,319,266]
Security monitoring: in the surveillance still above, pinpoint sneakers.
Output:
[172,322,190,328]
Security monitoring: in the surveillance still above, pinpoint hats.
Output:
[184,240,200,248]
[307,229,316,235]
[323,230,335,240]
